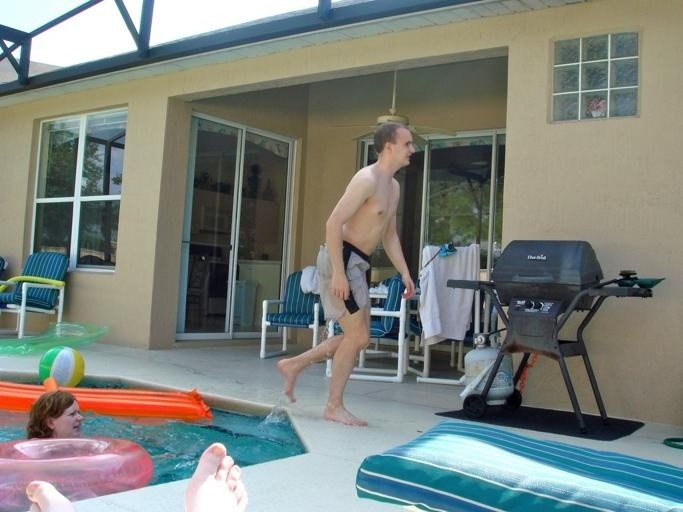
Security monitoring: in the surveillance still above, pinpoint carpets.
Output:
[433,401,645,442]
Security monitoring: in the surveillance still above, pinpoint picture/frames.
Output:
[199,204,228,233]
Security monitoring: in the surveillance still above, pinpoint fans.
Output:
[326,68,456,153]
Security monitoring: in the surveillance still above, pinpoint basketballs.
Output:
[38,346,84,387]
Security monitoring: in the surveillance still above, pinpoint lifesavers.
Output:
[0,438,153,511]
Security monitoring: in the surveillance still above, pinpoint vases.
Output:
[592,109,600,117]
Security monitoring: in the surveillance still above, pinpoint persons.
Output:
[25,441,249,512]
[276,121,415,426]
[27,389,84,437]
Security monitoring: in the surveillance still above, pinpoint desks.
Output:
[237,258,281,335]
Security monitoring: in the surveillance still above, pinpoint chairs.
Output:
[259,241,498,387]
[354,418,683,512]
[185,253,213,334]
[0,251,70,344]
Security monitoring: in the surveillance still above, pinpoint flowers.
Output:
[587,95,606,113]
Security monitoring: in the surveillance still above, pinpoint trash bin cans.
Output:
[234,280,258,328]
